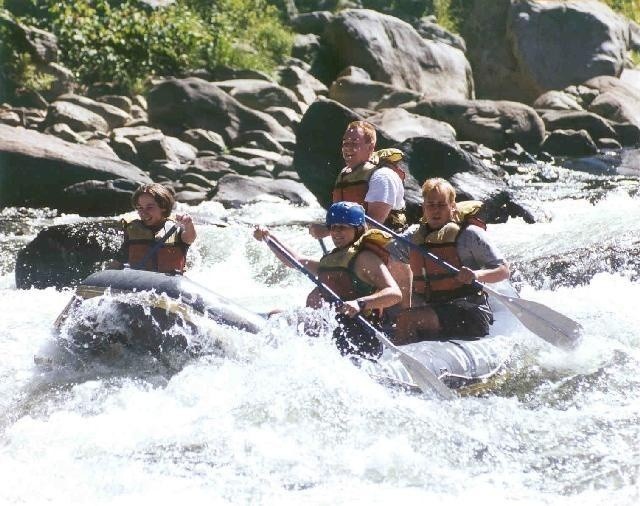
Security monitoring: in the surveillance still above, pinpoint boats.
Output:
[53,268,533,399]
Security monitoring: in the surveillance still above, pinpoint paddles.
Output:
[263,233,455,398]
[363,215,584,349]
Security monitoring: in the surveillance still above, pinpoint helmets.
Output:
[325,201,366,227]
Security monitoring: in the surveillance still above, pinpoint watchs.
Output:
[358,298,367,312]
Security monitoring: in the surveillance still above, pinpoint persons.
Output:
[309,119,411,238]
[384,176,512,343]
[253,200,403,363]
[105,181,198,276]
